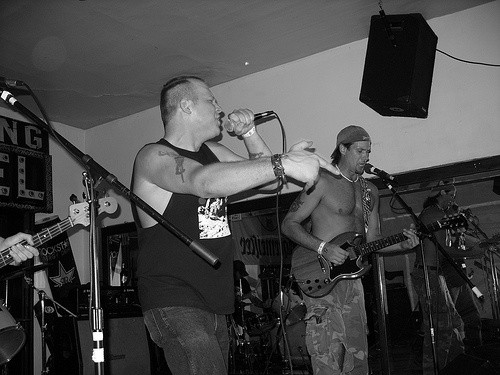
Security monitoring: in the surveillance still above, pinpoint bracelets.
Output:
[242,126,256,138]
[271,153,290,178]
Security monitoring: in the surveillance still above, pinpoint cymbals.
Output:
[234,301,256,306]
[258,267,290,279]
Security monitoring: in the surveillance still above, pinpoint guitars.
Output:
[0,189,118,270]
[291,212,469,298]
[437,203,467,290]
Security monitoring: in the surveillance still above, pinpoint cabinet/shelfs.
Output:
[54,311,157,375]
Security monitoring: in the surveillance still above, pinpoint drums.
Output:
[243,314,276,337]
[0,298,26,364]
[276,320,311,363]
[271,287,307,327]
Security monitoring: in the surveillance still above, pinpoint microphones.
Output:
[224,110,276,136]
[452,204,469,214]
[0,77,24,88]
[364,163,395,181]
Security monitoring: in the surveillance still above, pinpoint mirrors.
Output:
[100,222,137,290]
[377,170,499,375]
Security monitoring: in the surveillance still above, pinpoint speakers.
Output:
[359,12,438,119]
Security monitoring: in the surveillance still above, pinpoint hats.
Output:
[330,125,371,157]
[428,184,456,197]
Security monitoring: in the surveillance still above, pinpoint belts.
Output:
[414,265,437,270]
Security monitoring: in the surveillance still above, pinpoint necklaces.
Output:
[335,164,360,182]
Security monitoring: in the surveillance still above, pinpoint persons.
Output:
[234,261,252,298]
[130,75,340,375]
[318,241,326,254]
[0,232,39,266]
[410,175,483,375]
[281,125,419,375]
